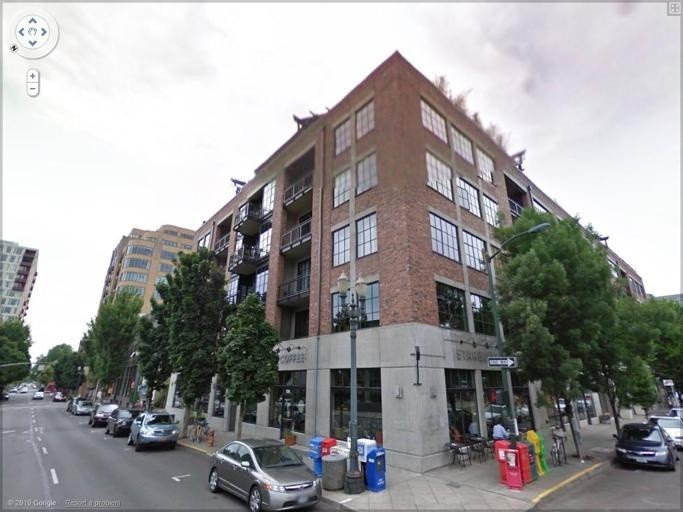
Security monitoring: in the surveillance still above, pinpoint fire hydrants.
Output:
[207,428,217,447]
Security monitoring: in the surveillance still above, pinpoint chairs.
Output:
[445,432,520,467]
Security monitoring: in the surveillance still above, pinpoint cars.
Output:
[67,396,182,454]
[206,438,323,511]
[273,396,305,422]
[3,381,53,402]
[53,391,67,401]
[612,422,679,471]
[518,393,593,416]
[648,416,683,449]
[482,404,508,424]
[668,407,683,420]
[446,407,478,432]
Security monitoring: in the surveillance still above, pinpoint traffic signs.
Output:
[486,355,517,368]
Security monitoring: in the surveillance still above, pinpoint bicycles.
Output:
[549,432,566,466]
[189,417,208,444]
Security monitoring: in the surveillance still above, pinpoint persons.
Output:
[468,419,479,437]
[492,420,510,439]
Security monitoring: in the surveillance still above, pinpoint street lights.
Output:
[333,269,369,494]
[480,221,553,440]
